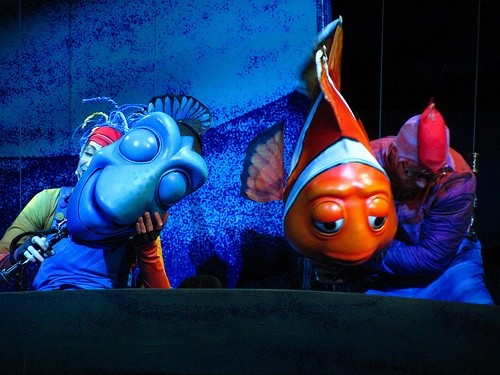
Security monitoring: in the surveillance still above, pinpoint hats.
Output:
[392,99,456,177]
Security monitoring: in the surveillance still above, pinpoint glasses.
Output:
[401,162,436,180]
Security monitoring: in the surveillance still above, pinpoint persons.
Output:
[0,97,171,289]
[358,98,496,306]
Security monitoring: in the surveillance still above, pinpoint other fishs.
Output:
[63,94,211,241]
[239,13,397,267]
